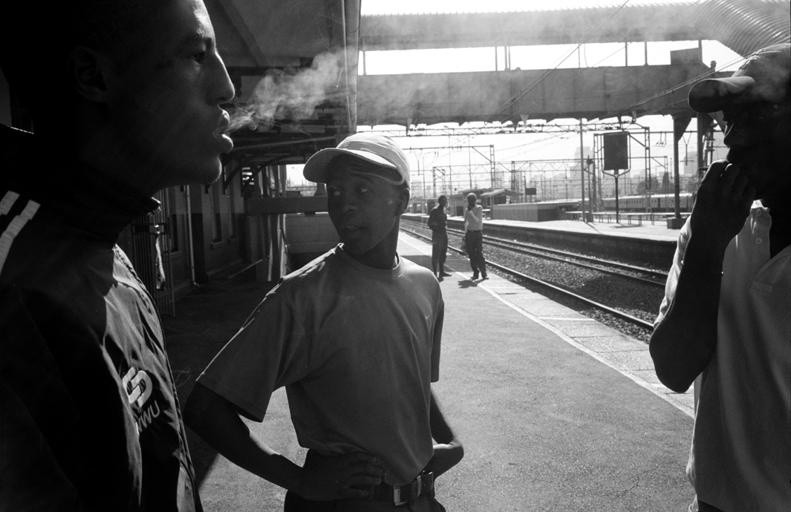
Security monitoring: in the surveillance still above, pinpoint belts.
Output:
[358,469,436,509]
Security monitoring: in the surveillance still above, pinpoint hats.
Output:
[688,42,790,114]
[302,131,411,190]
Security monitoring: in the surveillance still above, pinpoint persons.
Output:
[426,194,453,282]
[462,191,490,281]
[1,0,241,509]
[647,40,790,511]
[179,129,467,510]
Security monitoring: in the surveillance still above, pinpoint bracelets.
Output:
[677,257,726,281]
